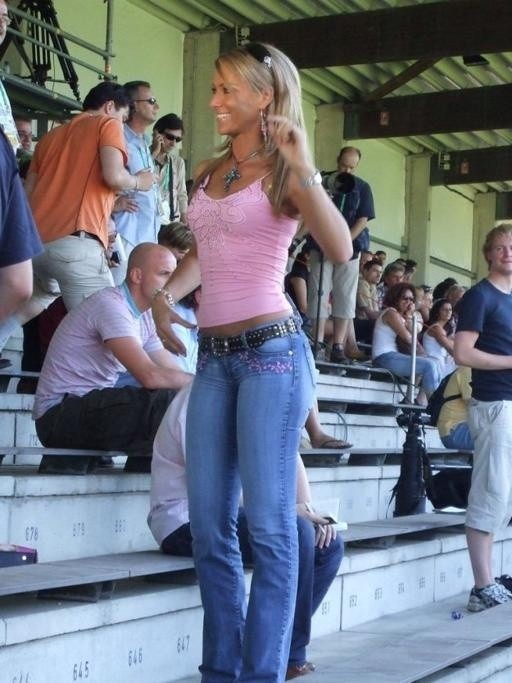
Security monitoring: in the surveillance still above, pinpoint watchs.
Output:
[300,169,322,190]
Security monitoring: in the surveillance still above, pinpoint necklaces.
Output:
[221,144,266,192]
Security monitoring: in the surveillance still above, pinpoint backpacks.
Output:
[426,369,463,426]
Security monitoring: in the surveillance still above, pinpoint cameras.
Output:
[319,172,356,198]
[391,413,433,519]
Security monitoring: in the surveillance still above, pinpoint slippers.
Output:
[318,439,353,448]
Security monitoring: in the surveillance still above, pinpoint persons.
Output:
[283,243,312,319]
[353,260,384,343]
[170,285,208,375]
[417,285,433,322]
[149,112,189,225]
[372,282,440,409]
[152,40,346,683]
[378,263,405,309]
[433,277,458,302]
[288,147,376,363]
[376,251,387,262]
[305,397,352,452]
[104,216,121,272]
[145,378,344,679]
[158,220,195,264]
[0,0,47,328]
[422,298,457,385]
[112,81,163,286]
[429,284,466,337]
[359,250,373,271]
[424,364,475,451]
[452,220,512,612]
[0,81,160,355]
[31,240,196,453]
[10,114,33,152]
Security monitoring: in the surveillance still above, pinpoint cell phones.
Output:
[153,129,165,154]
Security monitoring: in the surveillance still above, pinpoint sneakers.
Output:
[467,581,512,612]
[330,351,351,364]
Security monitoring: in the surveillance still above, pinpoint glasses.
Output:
[402,295,415,301]
[162,131,183,143]
[134,97,156,105]
[243,42,276,80]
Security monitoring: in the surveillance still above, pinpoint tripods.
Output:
[0,0,80,101]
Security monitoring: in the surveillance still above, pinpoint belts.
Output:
[72,228,97,240]
[198,318,295,359]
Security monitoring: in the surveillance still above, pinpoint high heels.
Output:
[346,346,370,362]
